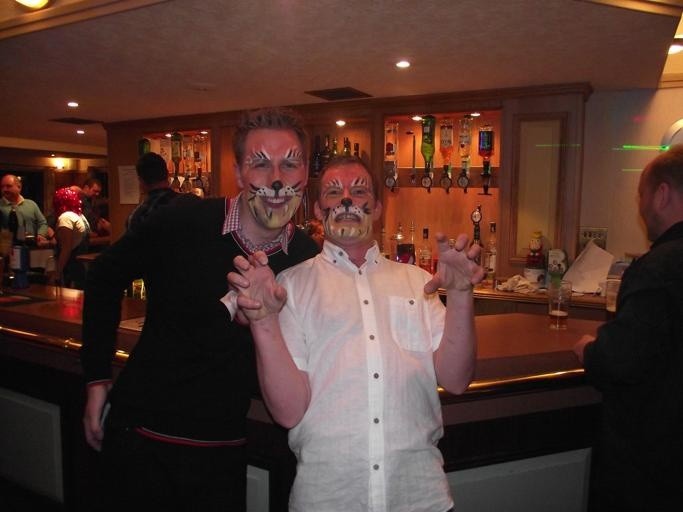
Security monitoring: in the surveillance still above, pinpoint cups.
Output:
[25,235,35,247]
[548,279,572,320]
[605,279,622,322]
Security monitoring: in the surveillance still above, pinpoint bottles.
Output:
[138,134,211,201]
[385,114,494,195]
[312,134,368,178]
[523,230,545,288]
[9,222,30,289]
[379,203,497,288]
[126,278,147,302]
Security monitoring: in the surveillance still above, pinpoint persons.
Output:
[568,146,681,512]
[226,152,486,510]
[0,175,109,286]
[126,151,203,233]
[77,106,323,510]
